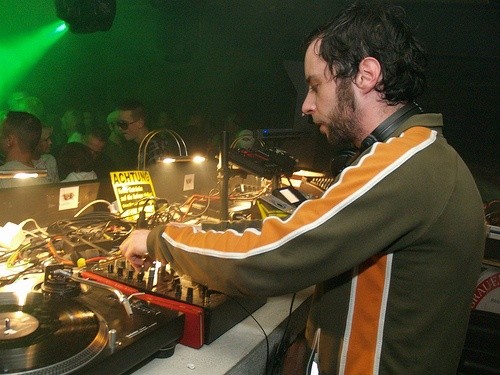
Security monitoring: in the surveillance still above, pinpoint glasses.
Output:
[117,120,140,129]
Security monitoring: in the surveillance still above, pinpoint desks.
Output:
[129,280,314,375]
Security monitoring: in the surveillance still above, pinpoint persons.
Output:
[118,5,486,375]
[0,96,212,184]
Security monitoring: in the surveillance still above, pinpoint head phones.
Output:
[330,101,424,183]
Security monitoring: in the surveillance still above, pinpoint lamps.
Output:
[55,0,116,33]
